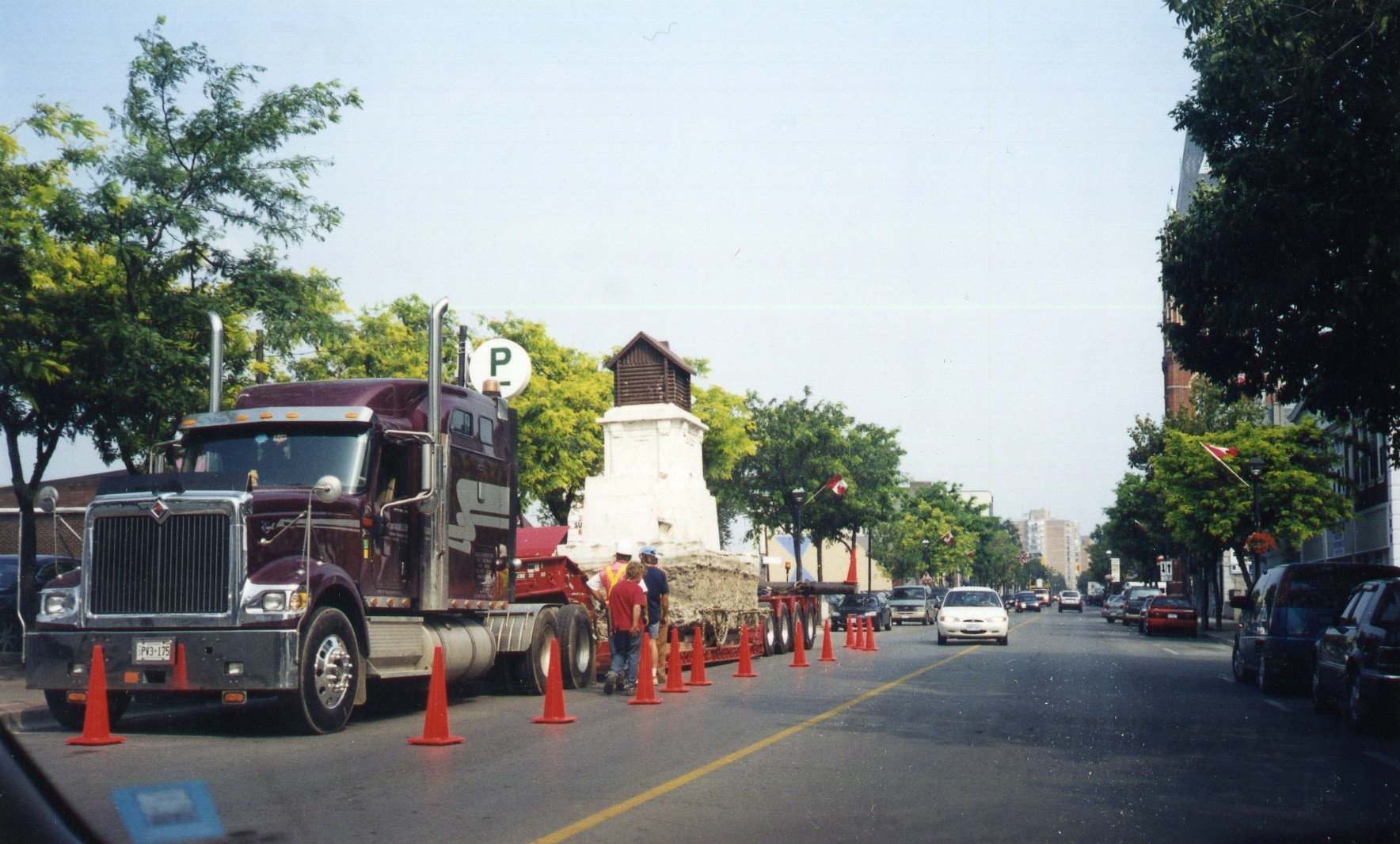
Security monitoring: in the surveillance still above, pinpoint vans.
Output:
[1231,561,1400,697]
[1034,589,1052,606]
[1057,589,1084,614]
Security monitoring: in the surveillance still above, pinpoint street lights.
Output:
[792,484,807,581]
[1105,549,1113,575]
[921,537,931,575]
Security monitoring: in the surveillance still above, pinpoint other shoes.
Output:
[652,677,658,686]
[603,674,617,695]
[615,673,624,691]
[625,686,636,696]
[657,675,667,683]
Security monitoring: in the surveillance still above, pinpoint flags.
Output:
[825,471,848,496]
[1198,439,1235,460]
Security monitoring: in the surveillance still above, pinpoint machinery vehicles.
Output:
[17,298,615,735]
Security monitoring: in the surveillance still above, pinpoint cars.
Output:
[0,553,84,649]
[932,586,1012,645]
[1014,591,1041,612]
[1100,585,1199,637]
[830,585,952,631]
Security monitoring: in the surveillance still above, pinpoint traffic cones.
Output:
[63,645,127,745]
[167,642,189,691]
[789,620,811,667]
[530,638,578,723]
[682,627,715,686]
[407,646,466,745]
[842,615,879,651]
[659,629,690,693]
[818,619,837,662]
[627,633,663,704]
[732,624,760,677]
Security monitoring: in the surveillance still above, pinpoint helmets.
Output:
[615,540,633,555]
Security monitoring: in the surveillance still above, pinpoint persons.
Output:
[980,593,997,607]
[637,544,671,683]
[603,560,646,695]
[953,594,964,606]
[585,541,648,690]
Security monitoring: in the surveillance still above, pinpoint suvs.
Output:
[1313,576,1400,730]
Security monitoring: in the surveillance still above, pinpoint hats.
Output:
[639,546,657,557]
